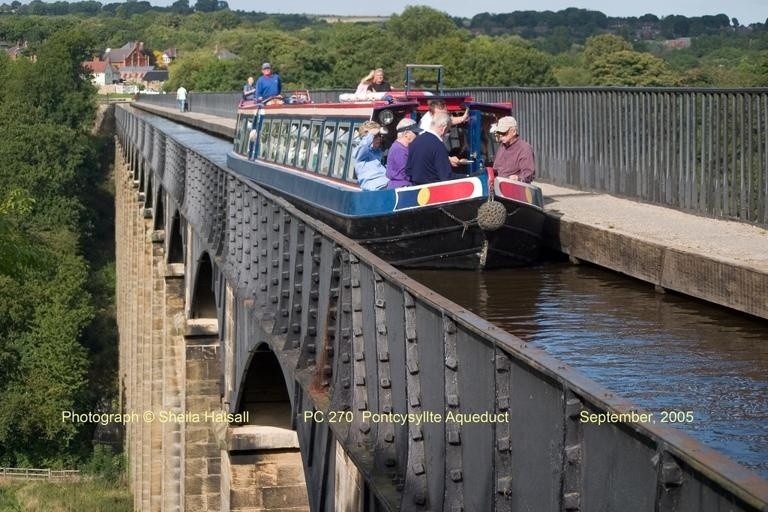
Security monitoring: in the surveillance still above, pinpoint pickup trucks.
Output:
[134,87,167,95]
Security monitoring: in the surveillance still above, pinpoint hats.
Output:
[262,62,271,69]
[493,115,518,134]
[396,122,424,134]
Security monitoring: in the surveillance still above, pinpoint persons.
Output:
[255,62,282,103]
[416,99,470,168]
[176,84,187,113]
[385,117,423,189]
[365,67,391,92]
[242,76,257,100]
[351,120,391,191]
[403,112,469,186]
[491,116,535,184]
[353,69,374,93]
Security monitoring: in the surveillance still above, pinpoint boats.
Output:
[226,91,547,269]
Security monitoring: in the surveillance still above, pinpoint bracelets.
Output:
[459,116,464,123]
